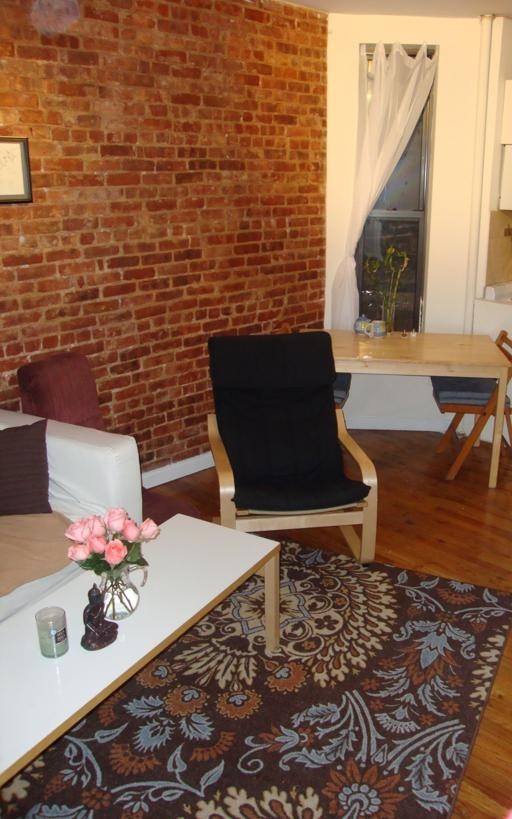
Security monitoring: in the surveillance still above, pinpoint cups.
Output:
[34,606,69,659]
[365,320,388,340]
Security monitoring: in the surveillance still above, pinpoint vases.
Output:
[97,566,138,622]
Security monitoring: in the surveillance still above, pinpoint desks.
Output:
[300,329,512,489]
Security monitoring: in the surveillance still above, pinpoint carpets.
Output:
[0,533,512,819]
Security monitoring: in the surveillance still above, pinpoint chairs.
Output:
[206,331,378,563]
[431,330,512,481]
[17,355,202,528]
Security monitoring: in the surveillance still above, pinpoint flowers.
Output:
[65,506,160,619]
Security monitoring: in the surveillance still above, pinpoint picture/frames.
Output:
[0,136,33,204]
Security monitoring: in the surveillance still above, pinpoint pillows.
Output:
[0,420,55,517]
